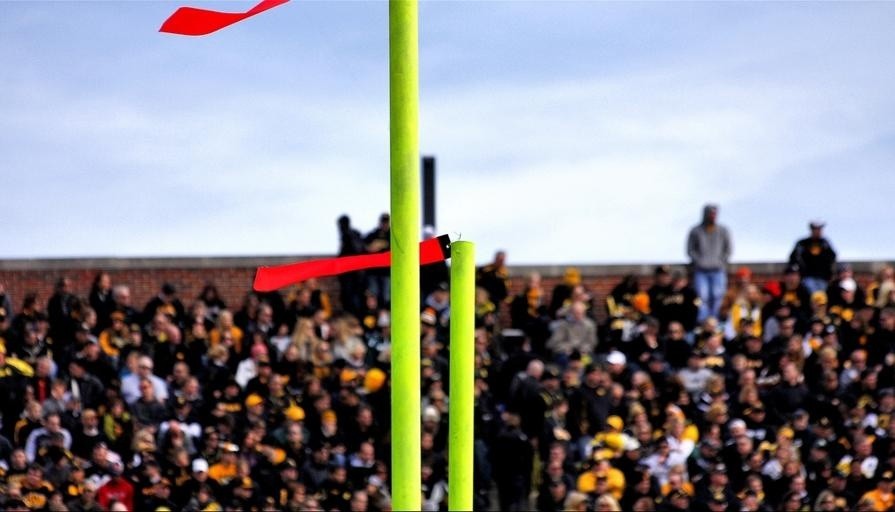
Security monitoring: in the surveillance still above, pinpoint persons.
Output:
[686,203,730,322]
[781,222,835,293]
[421,283,449,511]
[365,213,390,304]
[337,215,365,308]
[0,251,393,512]
[475,250,894,511]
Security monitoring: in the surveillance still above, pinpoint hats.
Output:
[193,458,206,472]
[606,351,625,364]
